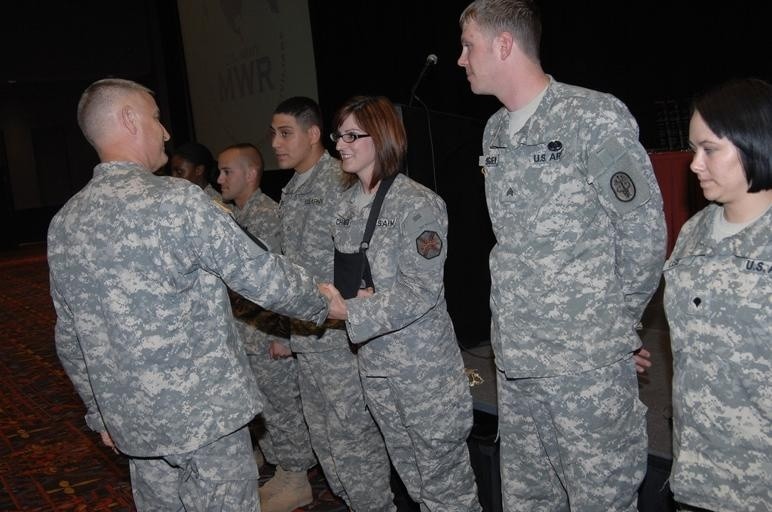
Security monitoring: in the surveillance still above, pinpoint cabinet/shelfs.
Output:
[646,150,706,260]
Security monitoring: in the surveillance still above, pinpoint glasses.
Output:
[330,130,374,143]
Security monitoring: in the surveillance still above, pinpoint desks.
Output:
[461,328,672,512]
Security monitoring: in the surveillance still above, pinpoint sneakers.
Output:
[257,464,289,508]
[259,470,314,512]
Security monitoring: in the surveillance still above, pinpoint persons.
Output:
[173,143,267,470]
[662,77,772,511]
[317,97,484,511]
[46,78,330,512]
[270,95,400,511]
[217,144,318,512]
[456,0,668,511]
